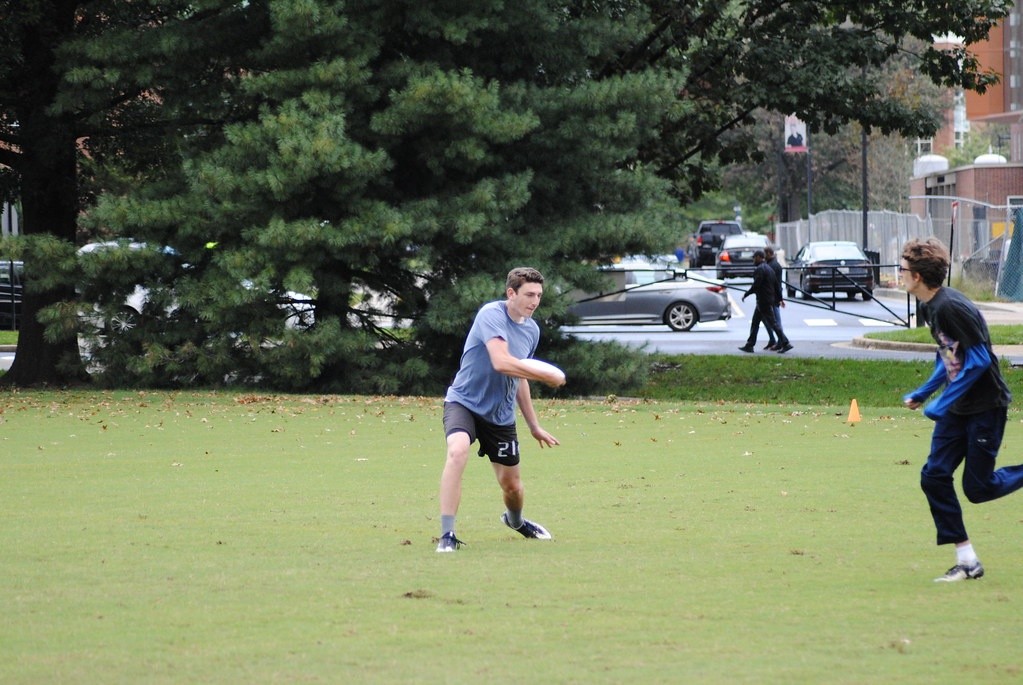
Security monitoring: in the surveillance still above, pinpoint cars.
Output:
[564,260,733,332]
[711,233,781,280]
[70,239,317,341]
[0,259,27,330]
[784,240,875,301]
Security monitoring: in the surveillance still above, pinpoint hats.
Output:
[751,251,765,259]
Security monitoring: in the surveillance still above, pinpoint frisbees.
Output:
[521,358,566,380]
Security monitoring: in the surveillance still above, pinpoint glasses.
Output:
[899,265,913,272]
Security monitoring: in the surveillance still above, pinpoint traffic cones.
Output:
[847,399,861,424]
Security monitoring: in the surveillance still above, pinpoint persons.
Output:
[433,265,566,551]
[787,124,803,148]
[899,237,1023,582]
[761,246,786,351]
[737,250,793,353]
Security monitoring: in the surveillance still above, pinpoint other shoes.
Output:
[764,342,774,349]
[770,342,782,350]
[777,344,793,353]
[739,343,754,352]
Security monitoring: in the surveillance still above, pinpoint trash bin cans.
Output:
[863,248,880,284]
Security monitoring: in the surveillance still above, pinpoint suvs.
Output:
[688,219,744,268]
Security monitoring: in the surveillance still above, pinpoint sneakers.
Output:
[500,511,551,540]
[935,562,985,583]
[437,531,467,552]
